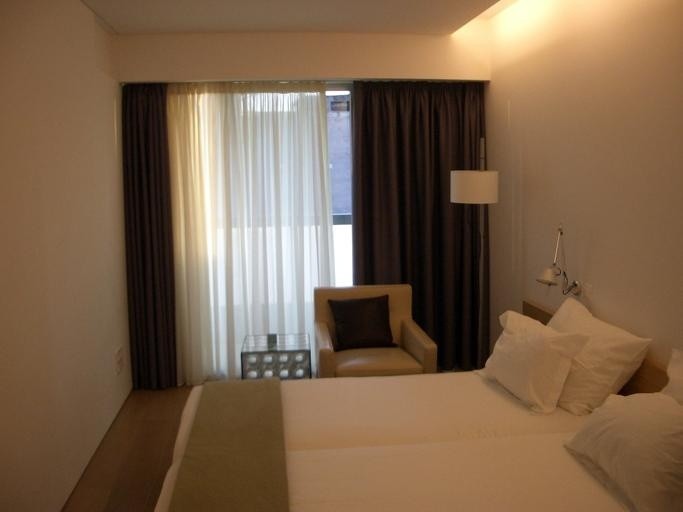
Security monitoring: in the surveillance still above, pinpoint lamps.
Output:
[449,135,501,369]
[536,223,583,297]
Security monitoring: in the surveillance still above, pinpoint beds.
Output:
[152,297,682,512]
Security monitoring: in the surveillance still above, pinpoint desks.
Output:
[240,334,311,379]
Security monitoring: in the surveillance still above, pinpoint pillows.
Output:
[480,311,590,413]
[545,297,652,416]
[567,390,681,511]
[664,349,679,401]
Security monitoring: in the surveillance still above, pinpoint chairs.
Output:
[312,283,437,379]
